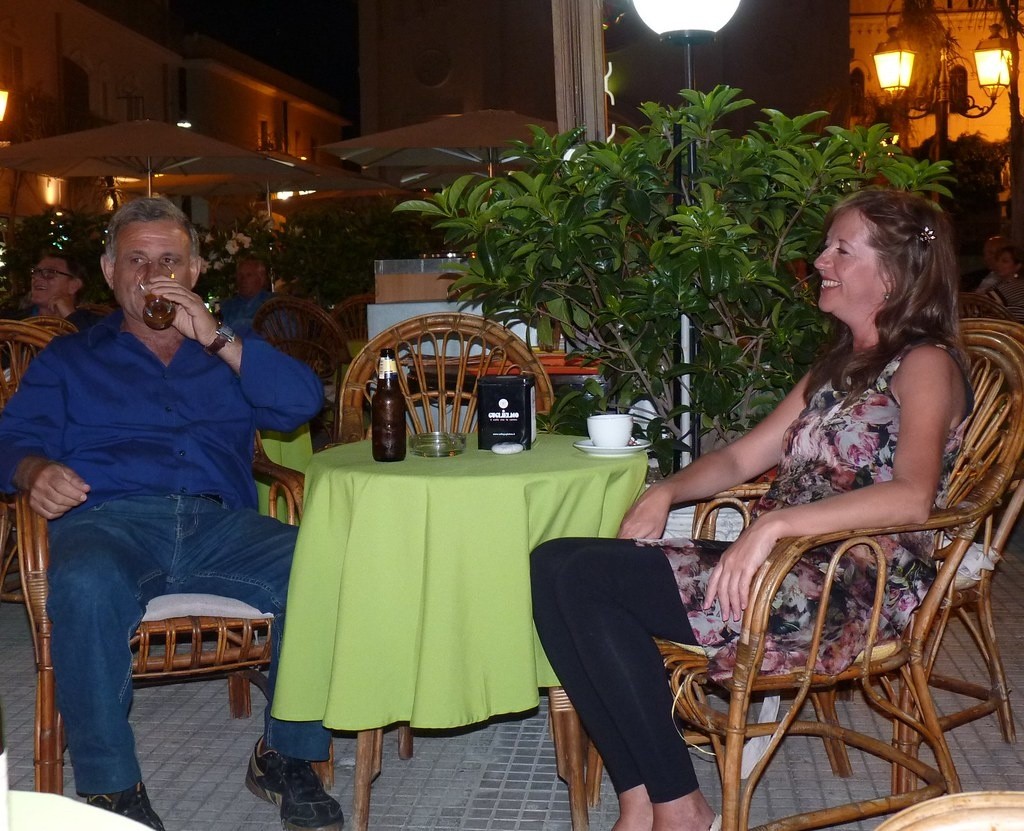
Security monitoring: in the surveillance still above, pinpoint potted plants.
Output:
[233,208,279,298]
[394,86,959,544]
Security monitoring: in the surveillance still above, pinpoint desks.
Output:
[268,431,651,831]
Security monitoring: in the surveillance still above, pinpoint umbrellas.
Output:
[312,107,558,199]
[205,141,436,215]
[0,118,319,198]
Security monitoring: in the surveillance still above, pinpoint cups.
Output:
[587,414,633,447]
[135,262,176,331]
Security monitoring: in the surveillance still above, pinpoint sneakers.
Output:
[245,736,344,831]
[87,782,165,831]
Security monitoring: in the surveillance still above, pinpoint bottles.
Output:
[371,349,407,463]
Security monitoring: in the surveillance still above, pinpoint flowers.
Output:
[195,219,251,293]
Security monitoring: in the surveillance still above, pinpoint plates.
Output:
[573,438,652,457]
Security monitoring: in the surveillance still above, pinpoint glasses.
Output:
[29,266,75,280]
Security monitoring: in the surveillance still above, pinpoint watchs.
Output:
[203,319,235,357]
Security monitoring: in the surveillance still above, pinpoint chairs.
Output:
[0,289,1024,831]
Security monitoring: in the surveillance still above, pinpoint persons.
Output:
[529,188,975,831]
[219,251,300,356]
[0,195,345,831]
[960,235,1024,325]
[0,254,105,381]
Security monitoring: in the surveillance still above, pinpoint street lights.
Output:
[873,23,1013,202]
[628,0,743,474]
